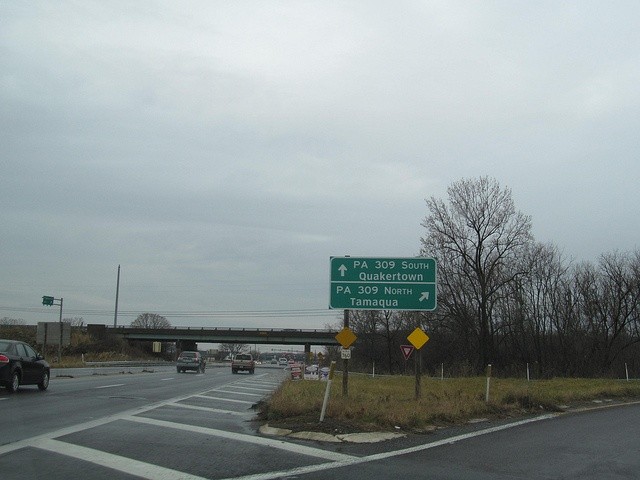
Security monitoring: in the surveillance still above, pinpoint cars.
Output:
[0,340,50,392]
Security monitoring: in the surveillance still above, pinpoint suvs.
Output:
[231,354,255,374]
[176,351,206,373]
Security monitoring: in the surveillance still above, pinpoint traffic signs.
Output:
[330,284,435,309]
[329,257,437,283]
